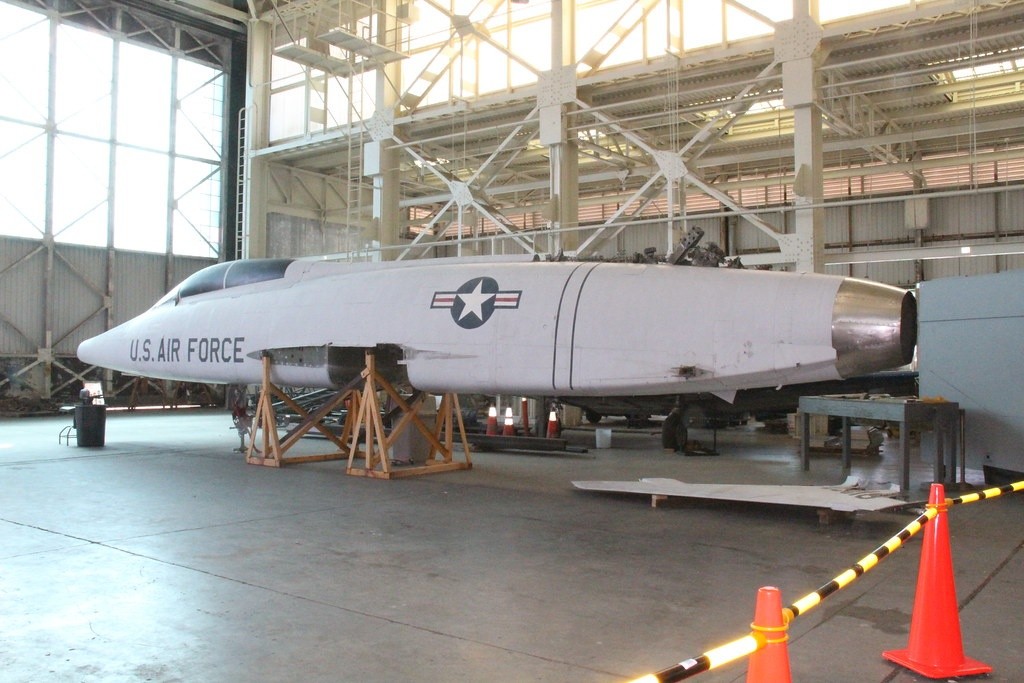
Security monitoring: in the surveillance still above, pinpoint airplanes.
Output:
[76,256,920,405]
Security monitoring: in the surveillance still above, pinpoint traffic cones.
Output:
[546,406,560,438]
[881,483,994,680]
[502,406,516,437]
[485,403,498,435]
[746,581,794,683]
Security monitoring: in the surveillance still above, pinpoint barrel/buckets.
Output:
[75,405,107,446]
[596,428,611,448]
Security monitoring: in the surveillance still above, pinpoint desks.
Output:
[798,392,960,497]
